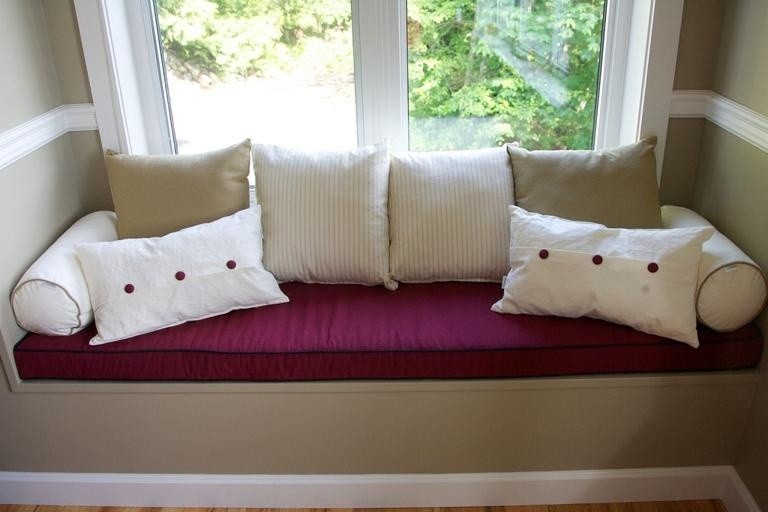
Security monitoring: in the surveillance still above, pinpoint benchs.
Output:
[0,280,768,507]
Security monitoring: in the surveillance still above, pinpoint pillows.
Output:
[72,205,287,345]
[388,150,514,283]
[11,212,120,337]
[491,205,713,345]
[503,138,663,229]
[249,144,394,292]
[104,140,250,242]
[657,200,767,333]
